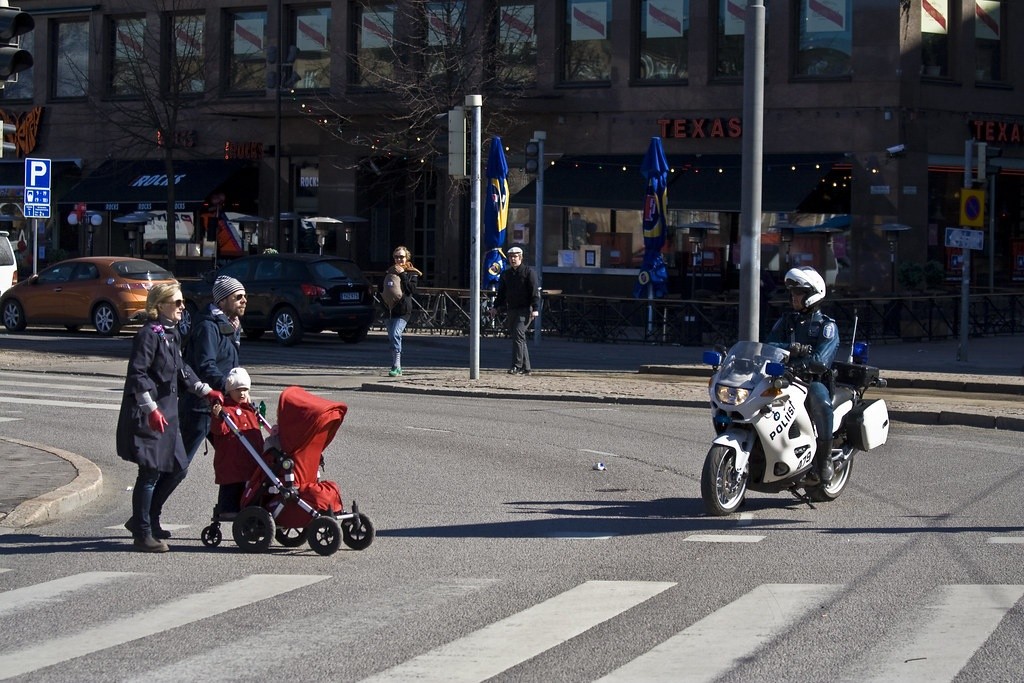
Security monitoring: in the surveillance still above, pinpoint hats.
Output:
[225,367,251,395]
[213,275,245,304]
[508,247,523,255]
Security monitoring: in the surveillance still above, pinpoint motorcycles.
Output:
[701,316,889,516]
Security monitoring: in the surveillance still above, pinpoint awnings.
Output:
[506,151,855,214]
[0,159,81,220]
[53,157,242,215]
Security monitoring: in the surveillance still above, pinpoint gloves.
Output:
[787,359,804,379]
[148,408,168,434]
[789,342,808,361]
[207,389,225,408]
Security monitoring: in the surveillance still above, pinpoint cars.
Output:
[177,253,376,347]
[0,256,177,336]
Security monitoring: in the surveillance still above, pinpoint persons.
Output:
[377,246,422,377]
[309,222,338,256]
[114,278,224,551]
[209,365,267,513]
[767,264,843,482]
[123,274,246,538]
[488,247,539,375]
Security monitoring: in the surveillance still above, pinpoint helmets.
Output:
[784,266,827,309]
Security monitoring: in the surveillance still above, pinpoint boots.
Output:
[811,437,834,484]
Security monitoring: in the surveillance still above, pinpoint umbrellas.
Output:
[632,137,667,332]
[482,136,510,329]
[302,216,344,225]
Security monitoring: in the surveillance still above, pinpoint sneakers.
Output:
[388,367,402,377]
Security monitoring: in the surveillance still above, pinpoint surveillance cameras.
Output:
[887,144,905,154]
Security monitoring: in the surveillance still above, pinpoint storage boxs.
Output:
[842,399,888,448]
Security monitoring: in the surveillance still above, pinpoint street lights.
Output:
[676,219,717,297]
[113,210,157,258]
[874,223,912,333]
[79,210,105,255]
[304,214,343,252]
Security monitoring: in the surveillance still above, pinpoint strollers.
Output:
[201,386,375,556]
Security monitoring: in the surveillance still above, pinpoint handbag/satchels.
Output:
[380,273,406,310]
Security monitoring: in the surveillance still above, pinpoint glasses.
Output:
[393,255,406,260]
[156,299,185,307]
[787,287,810,297]
[509,255,518,259]
[236,294,247,300]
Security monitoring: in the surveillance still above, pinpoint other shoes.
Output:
[219,512,238,519]
[151,527,171,538]
[134,530,169,552]
[515,369,532,376]
[124,517,135,532]
[508,364,520,374]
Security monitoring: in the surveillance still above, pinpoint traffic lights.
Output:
[978,142,1002,184]
[524,139,539,176]
[433,106,469,180]
[0,6,34,82]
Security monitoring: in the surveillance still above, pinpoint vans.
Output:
[0,231,17,297]
